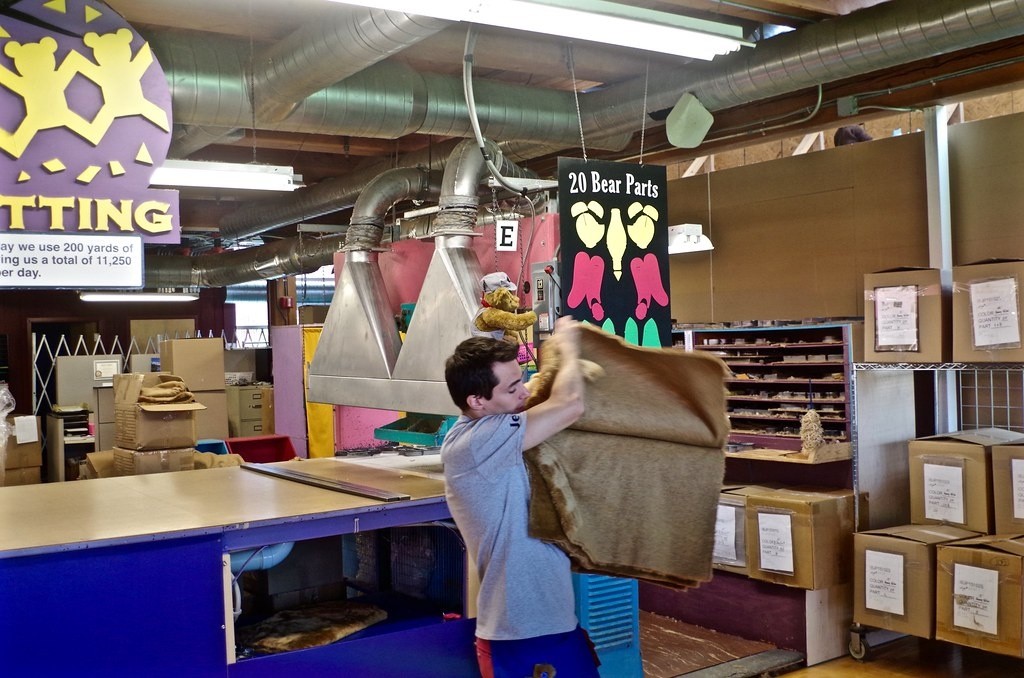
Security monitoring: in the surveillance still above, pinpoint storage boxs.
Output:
[863,266,951,364]
[712,480,870,590]
[951,258,1024,363]
[113,319,228,479]
[853,427,1024,659]
[0,414,43,488]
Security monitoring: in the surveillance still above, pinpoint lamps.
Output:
[148,0,307,191]
[79,291,200,302]
[327,0,758,63]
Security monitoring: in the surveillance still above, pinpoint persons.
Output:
[440,315,601,678]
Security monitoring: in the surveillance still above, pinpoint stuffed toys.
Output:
[473,271,537,343]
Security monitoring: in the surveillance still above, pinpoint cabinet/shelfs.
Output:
[848,363,1024,660]
[226,385,274,438]
[638,321,917,668]
[271,324,407,460]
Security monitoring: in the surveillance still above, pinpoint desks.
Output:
[47,414,95,482]
